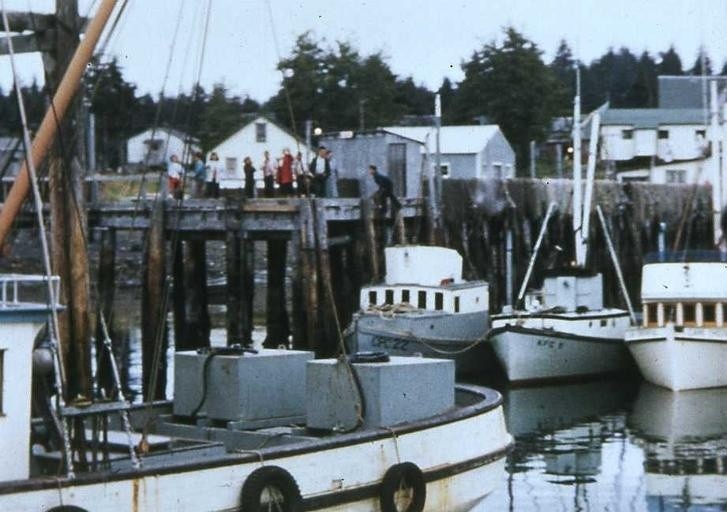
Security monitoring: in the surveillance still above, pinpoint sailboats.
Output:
[0,0,520,512]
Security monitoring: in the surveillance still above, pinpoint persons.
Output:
[204,152,225,199]
[308,146,332,196]
[276,148,294,192]
[260,151,278,197]
[167,154,186,200]
[190,152,207,196]
[294,151,314,192]
[242,156,256,198]
[324,150,339,197]
[368,162,403,214]
